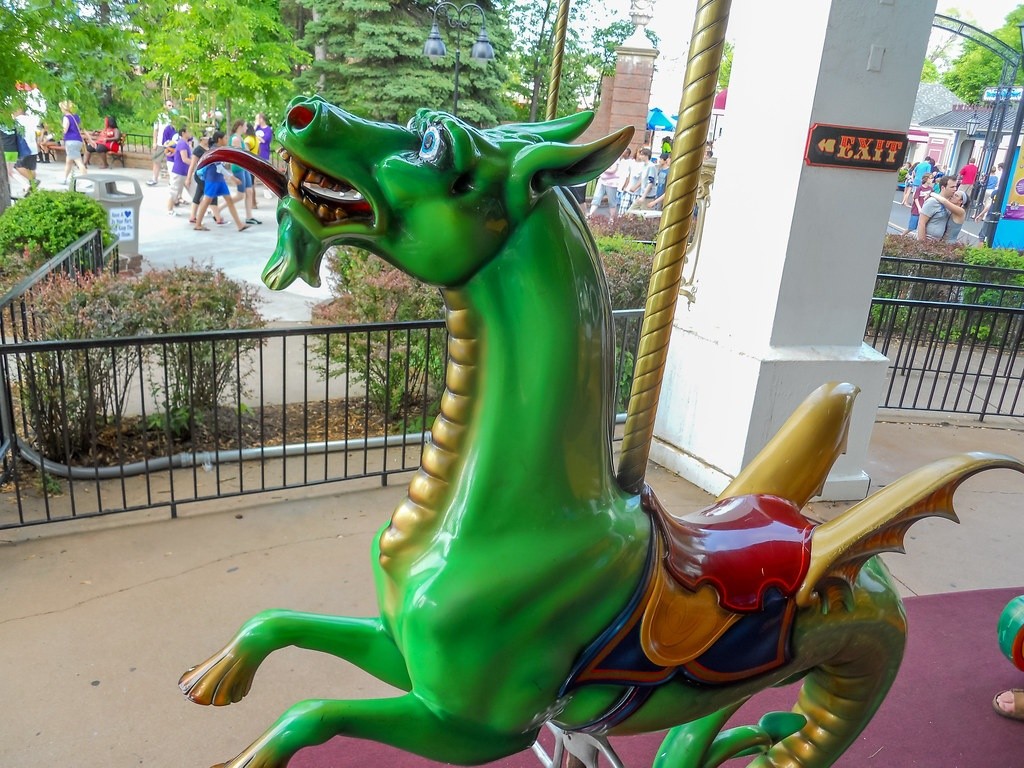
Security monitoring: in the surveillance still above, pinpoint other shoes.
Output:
[213,215,230,226]
[189,216,196,223]
[246,218,262,224]
[251,205,258,211]
[173,196,189,206]
[24,179,41,197]
[168,209,181,217]
[146,180,157,186]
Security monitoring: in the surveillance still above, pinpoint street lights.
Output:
[423,2,496,117]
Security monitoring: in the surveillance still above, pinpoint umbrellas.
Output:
[646,107,675,132]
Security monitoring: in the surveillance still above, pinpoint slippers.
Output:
[239,225,250,232]
[193,226,210,231]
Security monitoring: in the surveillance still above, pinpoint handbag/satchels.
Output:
[81,142,87,154]
[196,166,210,182]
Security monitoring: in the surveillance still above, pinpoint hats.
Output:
[59,100,71,111]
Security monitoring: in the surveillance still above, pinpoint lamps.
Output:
[966,110,985,139]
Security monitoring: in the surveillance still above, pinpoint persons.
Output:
[901,156,1004,243]
[78,116,121,169]
[146,100,272,231]
[58,101,97,188]
[992,687,1024,719]
[568,136,673,222]
[0,107,60,201]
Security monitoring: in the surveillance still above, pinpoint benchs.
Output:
[47,133,125,166]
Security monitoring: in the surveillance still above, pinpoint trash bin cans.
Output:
[69,174,144,256]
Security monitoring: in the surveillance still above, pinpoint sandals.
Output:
[993,688,1024,720]
